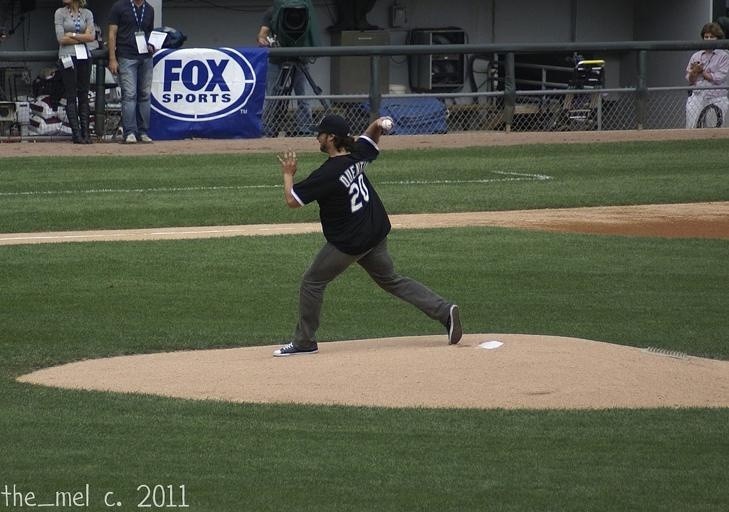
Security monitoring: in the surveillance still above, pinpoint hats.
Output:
[309,115,351,137]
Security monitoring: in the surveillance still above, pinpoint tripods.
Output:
[262,41,333,128]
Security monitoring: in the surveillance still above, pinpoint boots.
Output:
[78,103,92,143]
[65,103,86,144]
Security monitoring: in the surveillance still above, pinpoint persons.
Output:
[270,114,465,357]
[682,22,729,129]
[107,0,156,144]
[53,1,97,145]
[256,0,321,138]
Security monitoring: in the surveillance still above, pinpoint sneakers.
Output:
[124,133,137,143]
[446,305,462,345]
[137,133,152,143]
[273,342,319,356]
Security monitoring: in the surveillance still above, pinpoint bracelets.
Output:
[699,69,703,75]
[72,33,77,38]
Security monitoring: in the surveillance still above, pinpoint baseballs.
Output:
[381,119,393,131]
